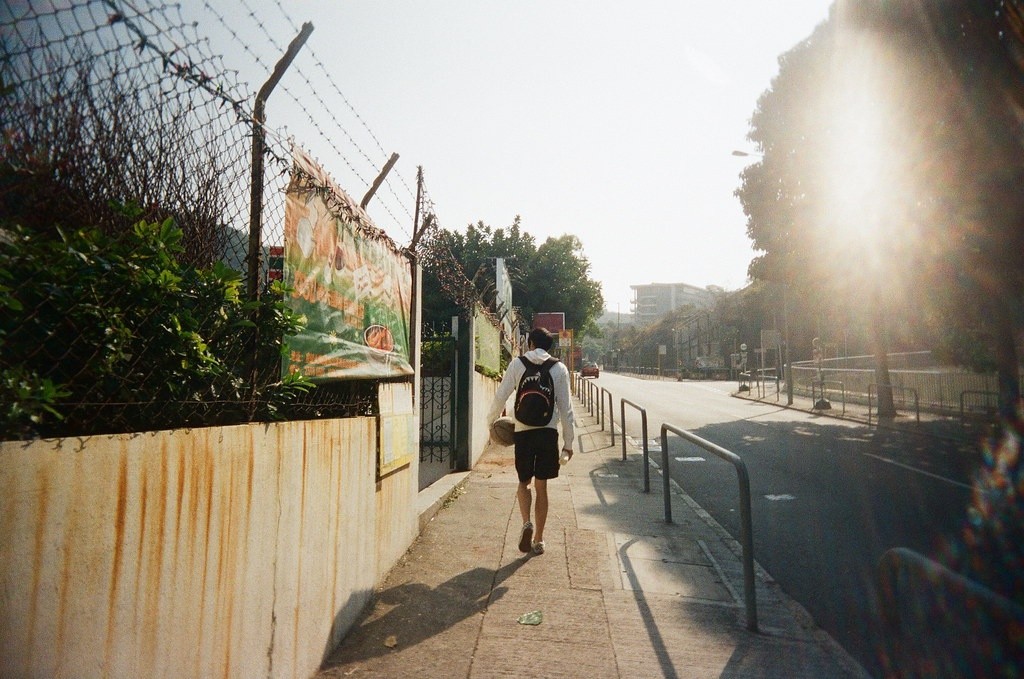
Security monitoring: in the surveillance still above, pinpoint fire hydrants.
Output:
[678,373,683,381]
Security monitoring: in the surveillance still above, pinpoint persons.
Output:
[488,327,574,555]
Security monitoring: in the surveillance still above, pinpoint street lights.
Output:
[672,329,678,375]
[617,301,620,331]
[730,151,794,407]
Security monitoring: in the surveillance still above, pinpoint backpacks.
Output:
[513,355,561,427]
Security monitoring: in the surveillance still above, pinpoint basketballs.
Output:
[489,416,515,446]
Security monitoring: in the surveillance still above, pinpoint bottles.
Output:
[558,451,569,465]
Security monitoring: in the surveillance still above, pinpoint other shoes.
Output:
[518,521,533,553]
[531,539,545,554]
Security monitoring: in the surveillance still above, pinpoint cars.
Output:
[581,363,599,378]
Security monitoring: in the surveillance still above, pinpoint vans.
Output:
[567,355,587,372]
